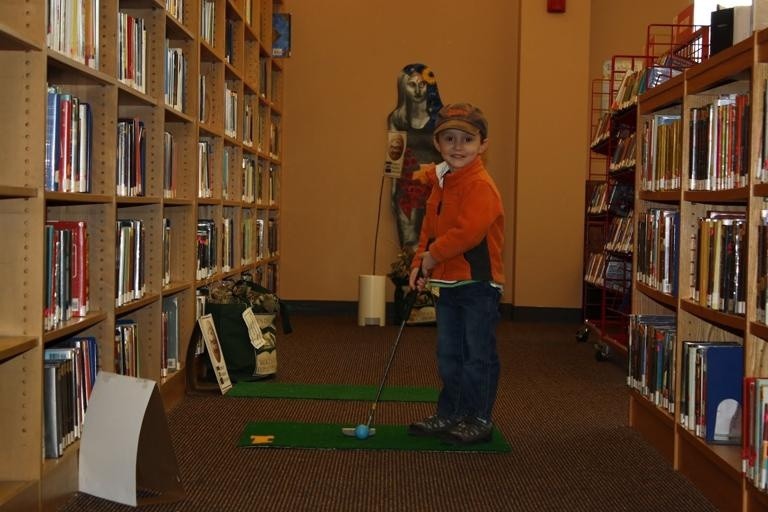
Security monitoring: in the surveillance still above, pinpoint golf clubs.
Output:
[340,238,434,438]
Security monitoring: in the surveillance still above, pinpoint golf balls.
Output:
[355,425,369,439]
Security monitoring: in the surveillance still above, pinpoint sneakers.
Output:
[407,412,466,433]
[441,416,493,444]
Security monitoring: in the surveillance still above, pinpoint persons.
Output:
[406,101,505,445]
[387,63,447,308]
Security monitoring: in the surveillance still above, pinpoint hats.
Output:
[434,102,488,138]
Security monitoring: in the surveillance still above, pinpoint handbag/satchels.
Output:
[203,300,278,383]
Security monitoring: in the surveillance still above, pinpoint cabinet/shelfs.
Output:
[576,24,766,507]
[1,0,283,507]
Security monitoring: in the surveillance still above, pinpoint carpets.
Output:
[227,380,513,455]
